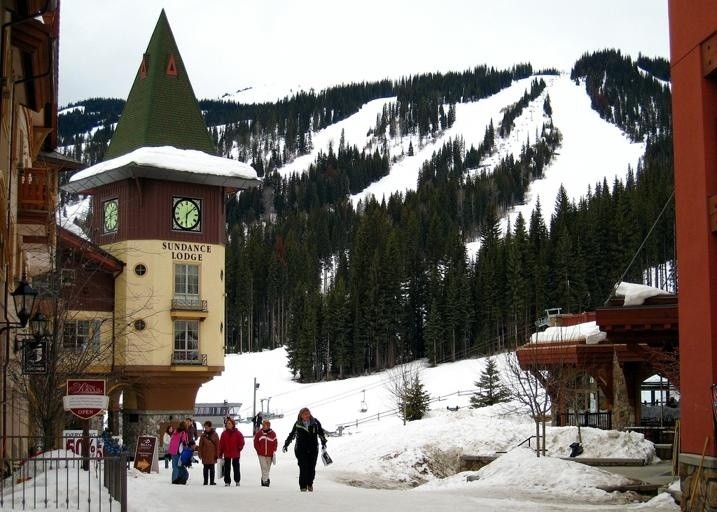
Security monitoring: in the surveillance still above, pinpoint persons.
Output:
[163,418,199,486]
[282,408,328,493]
[197,421,219,486]
[253,420,278,486]
[252,411,263,436]
[224,414,232,429]
[218,420,245,487]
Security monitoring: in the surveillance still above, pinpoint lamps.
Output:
[13,304,49,354]
[0,269,39,340]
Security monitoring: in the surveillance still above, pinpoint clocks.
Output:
[102,197,120,234]
[172,196,202,233]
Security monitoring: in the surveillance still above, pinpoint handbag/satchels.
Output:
[178,441,184,454]
